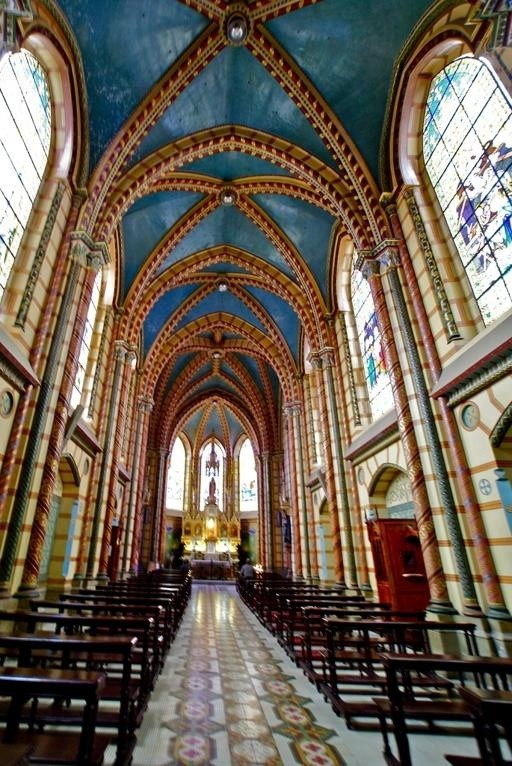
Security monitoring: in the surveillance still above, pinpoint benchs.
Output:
[0,571,193,766]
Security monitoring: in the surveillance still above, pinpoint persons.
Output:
[241,560,255,578]
